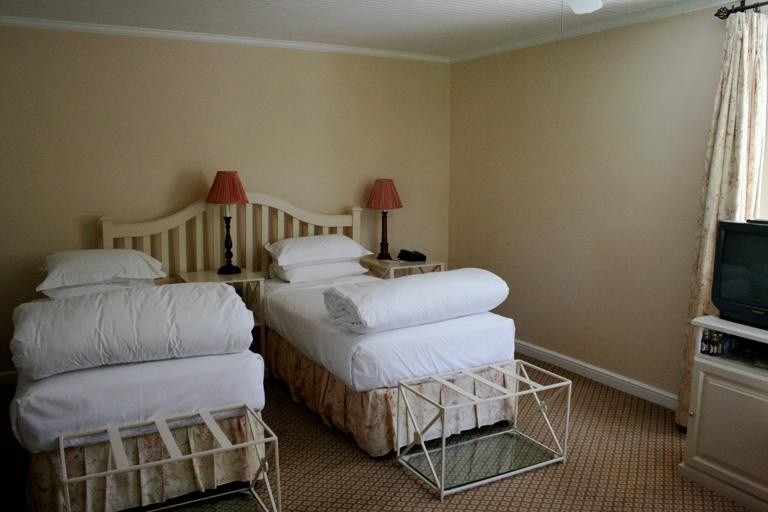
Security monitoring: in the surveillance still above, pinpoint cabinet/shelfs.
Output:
[670,304,768,511]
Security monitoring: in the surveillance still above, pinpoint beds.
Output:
[238,180,524,464]
[9,183,280,512]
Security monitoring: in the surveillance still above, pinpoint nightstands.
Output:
[176,267,266,384]
[363,254,448,280]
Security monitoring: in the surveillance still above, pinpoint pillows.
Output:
[43,279,160,303]
[268,260,371,284]
[261,231,375,272]
[33,246,168,295]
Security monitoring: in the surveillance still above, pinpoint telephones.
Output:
[397,249,426,262]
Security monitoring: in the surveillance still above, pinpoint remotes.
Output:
[747,218,768,224]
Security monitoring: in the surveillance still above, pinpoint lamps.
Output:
[364,177,406,262]
[204,167,253,275]
[563,1,608,17]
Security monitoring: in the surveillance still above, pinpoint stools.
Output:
[389,357,577,503]
[51,400,290,511]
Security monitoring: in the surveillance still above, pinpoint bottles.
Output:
[701,330,737,354]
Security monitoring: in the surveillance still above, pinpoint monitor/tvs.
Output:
[710,221,768,329]
